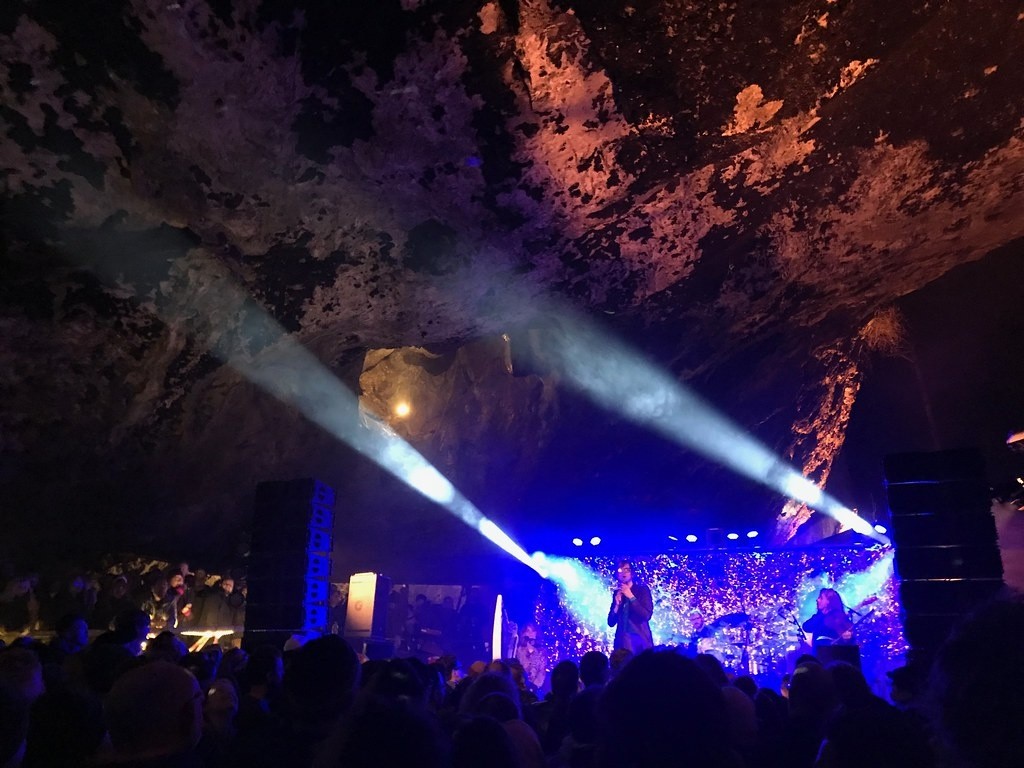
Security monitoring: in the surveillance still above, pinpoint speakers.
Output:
[344,572,392,639]
[238,476,334,652]
[879,451,1007,666]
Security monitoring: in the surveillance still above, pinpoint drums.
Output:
[743,646,771,674]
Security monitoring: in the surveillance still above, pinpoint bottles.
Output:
[331,622,338,634]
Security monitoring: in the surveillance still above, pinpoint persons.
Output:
[0,559,1024,768]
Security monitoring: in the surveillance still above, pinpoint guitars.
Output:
[817,609,875,647]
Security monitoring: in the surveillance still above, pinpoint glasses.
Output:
[617,568,631,573]
